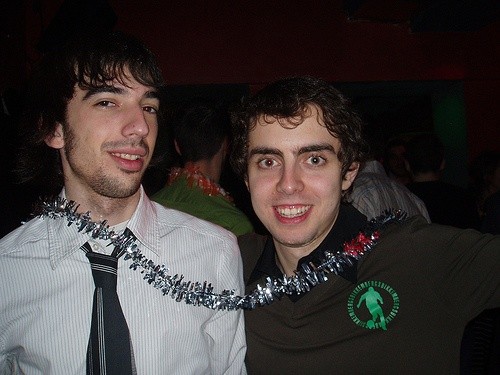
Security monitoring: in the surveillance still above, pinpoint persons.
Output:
[227,75,500,375]
[0,27,252,375]
[153,102,500,240]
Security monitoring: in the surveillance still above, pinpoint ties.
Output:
[82,227,137,375]
